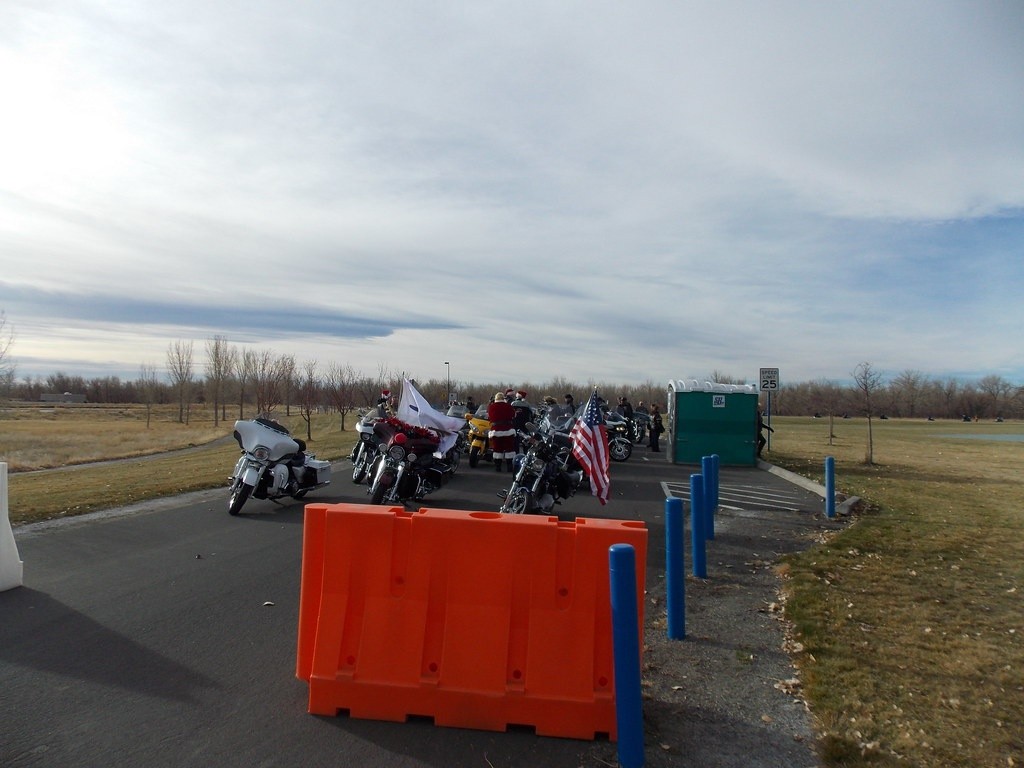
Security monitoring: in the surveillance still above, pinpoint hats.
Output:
[621,397,627,401]
[505,389,515,395]
[382,390,391,399]
[516,391,526,399]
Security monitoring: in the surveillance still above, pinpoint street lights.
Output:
[445,361,450,407]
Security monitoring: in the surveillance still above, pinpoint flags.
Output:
[396,377,465,455]
[568,389,611,506]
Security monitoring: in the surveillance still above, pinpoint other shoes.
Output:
[647,444,652,447]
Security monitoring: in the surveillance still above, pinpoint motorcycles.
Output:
[497,414,594,515]
[346,395,474,512]
[465,402,537,468]
[533,400,646,462]
[227,417,332,515]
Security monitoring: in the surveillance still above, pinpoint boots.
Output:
[507,458,513,473]
[494,459,502,472]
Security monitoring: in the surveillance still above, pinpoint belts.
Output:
[492,421,512,426]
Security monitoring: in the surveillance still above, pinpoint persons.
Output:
[758,403,774,461]
[381,390,399,411]
[541,395,558,419]
[563,394,574,416]
[465,396,475,411]
[487,388,533,473]
[636,401,649,414]
[646,403,662,452]
[616,396,635,448]
